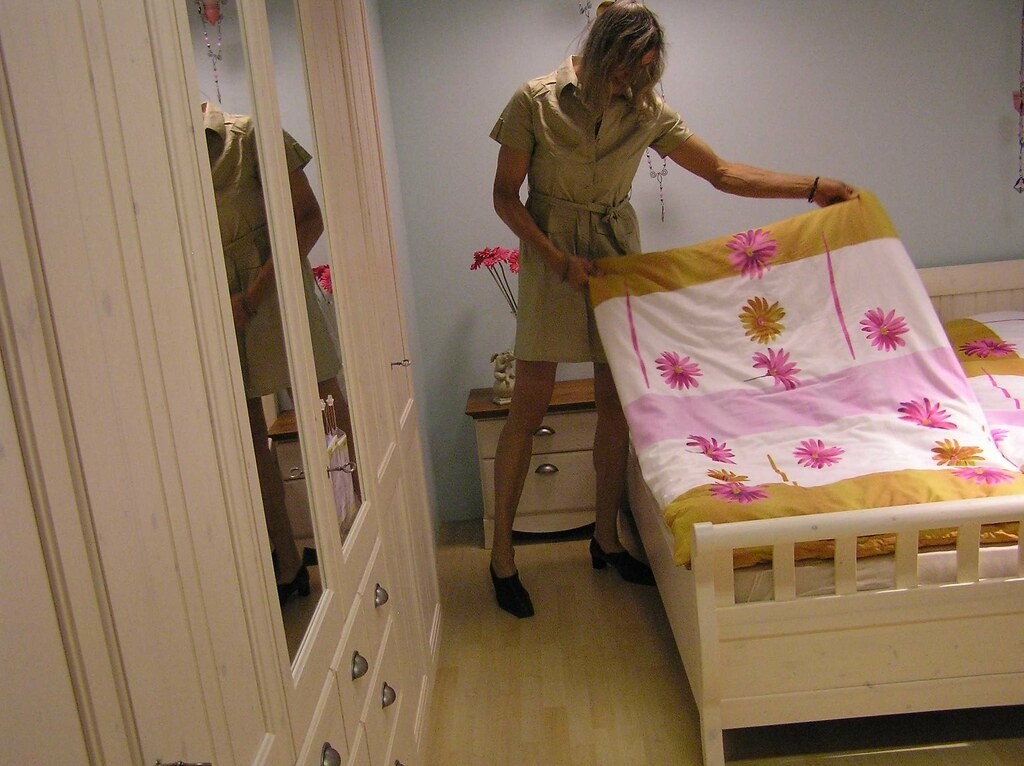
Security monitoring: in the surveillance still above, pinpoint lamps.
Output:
[195,0,227,104]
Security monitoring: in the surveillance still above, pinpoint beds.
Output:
[629,259,1024,766]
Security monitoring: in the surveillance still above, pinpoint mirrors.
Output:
[186,1,327,671]
[266,1,363,550]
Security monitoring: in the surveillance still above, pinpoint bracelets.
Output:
[240,292,256,314]
[808,176,819,203]
[560,256,569,284]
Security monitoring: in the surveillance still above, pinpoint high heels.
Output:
[277,565,310,607]
[590,536,656,585]
[490,560,535,618]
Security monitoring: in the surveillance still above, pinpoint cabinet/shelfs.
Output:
[2,1,443,766]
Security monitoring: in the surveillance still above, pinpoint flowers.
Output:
[471,246,519,316]
[312,265,333,315]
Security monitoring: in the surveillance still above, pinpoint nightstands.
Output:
[465,379,597,550]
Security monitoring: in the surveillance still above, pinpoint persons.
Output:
[200,101,361,607]
[488,0,861,618]
